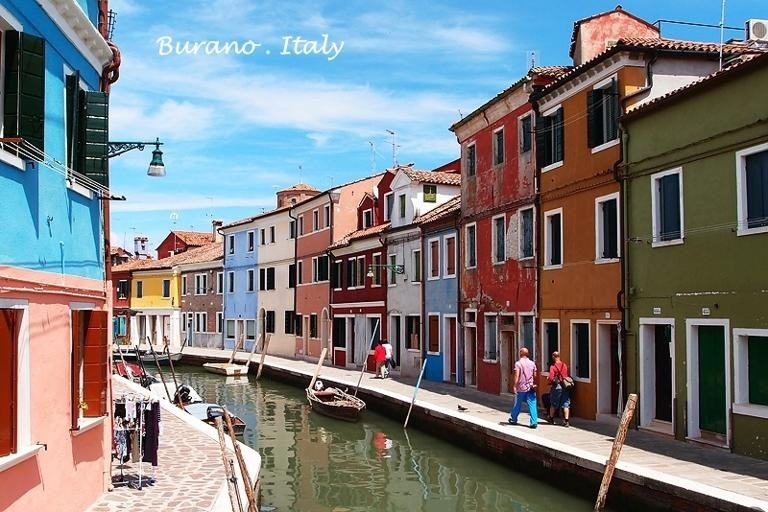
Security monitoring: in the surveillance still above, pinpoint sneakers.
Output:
[508,419,537,429]
[540,415,570,427]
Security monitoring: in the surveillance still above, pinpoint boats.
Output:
[145,383,245,436]
[202,363,249,376]
[113,349,183,383]
[307,386,366,419]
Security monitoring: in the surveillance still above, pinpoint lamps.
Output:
[366,263,404,278]
[107,137,167,177]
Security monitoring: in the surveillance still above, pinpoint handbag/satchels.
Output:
[561,376,575,391]
[552,376,558,388]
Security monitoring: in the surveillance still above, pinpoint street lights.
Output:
[383,128,395,166]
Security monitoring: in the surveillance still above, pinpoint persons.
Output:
[542,350,572,428]
[373,339,387,379]
[381,338,393,378]
[509,347,540,428]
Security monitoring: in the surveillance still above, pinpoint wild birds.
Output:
[458,407,468,412]
[458,404,461,408]
